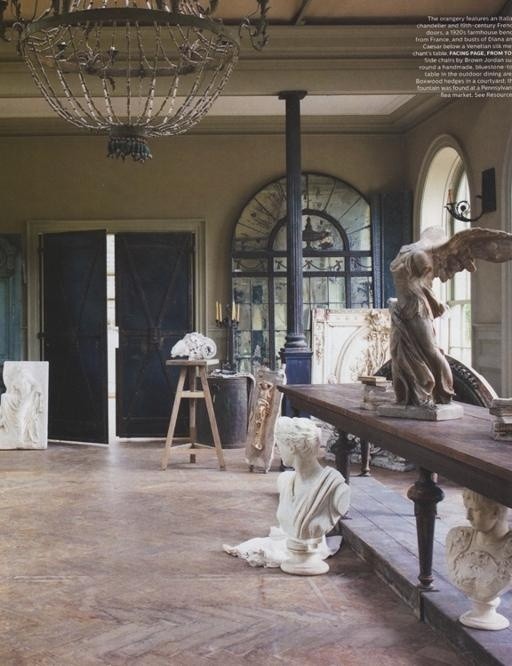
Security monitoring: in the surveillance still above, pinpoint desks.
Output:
[276,379,512,590]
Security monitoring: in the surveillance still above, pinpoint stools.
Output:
[161,359,226,471]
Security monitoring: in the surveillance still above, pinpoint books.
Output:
[358,375,387,383]
[360,380,393,387]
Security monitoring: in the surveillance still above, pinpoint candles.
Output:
[448,189,453,203]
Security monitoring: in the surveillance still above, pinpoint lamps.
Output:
[0,0,272,164]
[215,299,241,375]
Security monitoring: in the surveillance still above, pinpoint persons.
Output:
[272,415,352,576]
[387,246,453,406]
[445,487,511,600]
[255,380,273,434]
